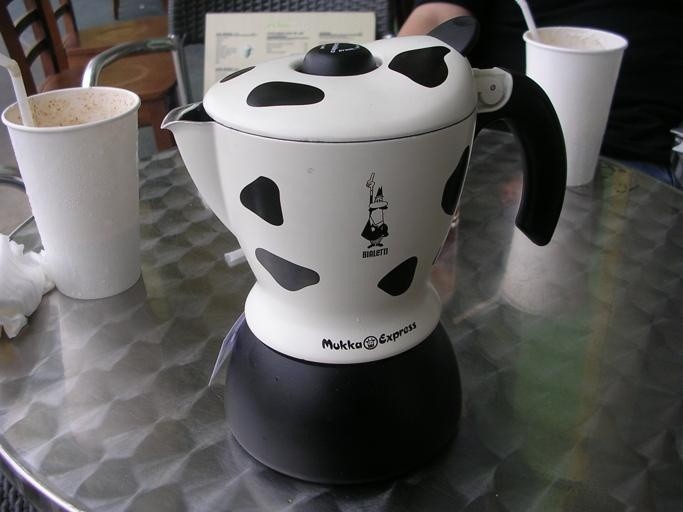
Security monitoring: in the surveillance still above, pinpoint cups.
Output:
[522,27,628,189]
[1,87,142,300]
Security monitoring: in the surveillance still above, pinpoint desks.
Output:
[0,130,681,510]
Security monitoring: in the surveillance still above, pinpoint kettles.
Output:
[161,36,568,492]
